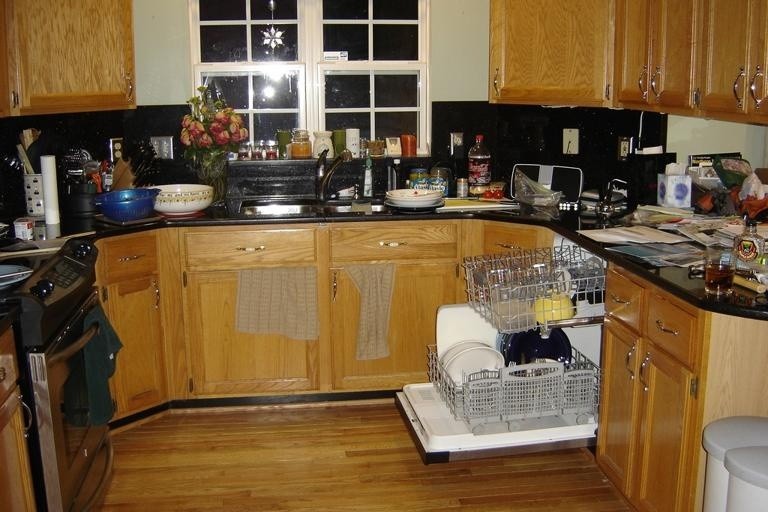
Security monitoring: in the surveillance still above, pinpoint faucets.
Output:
[314,147,352,201]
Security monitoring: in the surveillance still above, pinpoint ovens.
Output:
[15,287,115,510]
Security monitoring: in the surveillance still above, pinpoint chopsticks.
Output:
[16,144,34,174]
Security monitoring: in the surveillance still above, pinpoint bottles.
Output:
[467,135,492,200]
[363,159,374,200]
[388,158,407,190]
[730,218,765,276]
[457,178,467,197]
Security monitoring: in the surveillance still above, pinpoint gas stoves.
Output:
[0,236,100,350]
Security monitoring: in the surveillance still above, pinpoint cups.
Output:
[238,139,279,160]
[705,246,739,298]
[22,174,45,217]
[292,127,365,158]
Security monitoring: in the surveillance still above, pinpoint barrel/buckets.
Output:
[469,135,492,183]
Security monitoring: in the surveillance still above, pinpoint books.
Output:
[577,202,766,267]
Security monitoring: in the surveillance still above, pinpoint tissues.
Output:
[656,162,691,208]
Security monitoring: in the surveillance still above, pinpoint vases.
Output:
[195,149,228,207]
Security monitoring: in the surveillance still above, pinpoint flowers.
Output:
[177,85,248,205]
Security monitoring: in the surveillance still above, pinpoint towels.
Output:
[81,309,121,423]
[344,261,397,362]
[235,267,318,337]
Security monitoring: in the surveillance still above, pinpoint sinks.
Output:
[322,198,393,216]
[238,199,321,218]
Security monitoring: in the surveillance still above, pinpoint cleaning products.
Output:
[359,148,376,198]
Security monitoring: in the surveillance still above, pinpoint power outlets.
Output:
[109,137,123,162]
[617,136,633,161]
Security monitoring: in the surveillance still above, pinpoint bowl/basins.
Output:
[90,186,163,220]
[146,185,216,216]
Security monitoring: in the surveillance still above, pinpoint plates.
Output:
[384,188,446,212]
[502,322,572,374]
[437,340,504,386]
[1,263,36,292]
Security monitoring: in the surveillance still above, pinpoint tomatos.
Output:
[493,190,503,199]
[483,191,492,199]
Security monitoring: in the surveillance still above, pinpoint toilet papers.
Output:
[45,224,59,238]
[39,154,61,225]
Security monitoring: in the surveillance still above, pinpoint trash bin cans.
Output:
[702,415,768,512]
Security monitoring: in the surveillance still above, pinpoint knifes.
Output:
[120,135,160,187]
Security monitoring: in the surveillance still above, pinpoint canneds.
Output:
[428,167,448,198]
[409,168,428,189]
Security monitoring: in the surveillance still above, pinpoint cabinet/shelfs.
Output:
[181,225,330,403]
[478,216,549,260]
[0,298,47,512]
[699,3,767,126]
[616,2,698,115]
[595,259,766,512]
[329,221,470,399]
[2,2,138,118]
[490,0,613,105]
[94,228,162,436]
